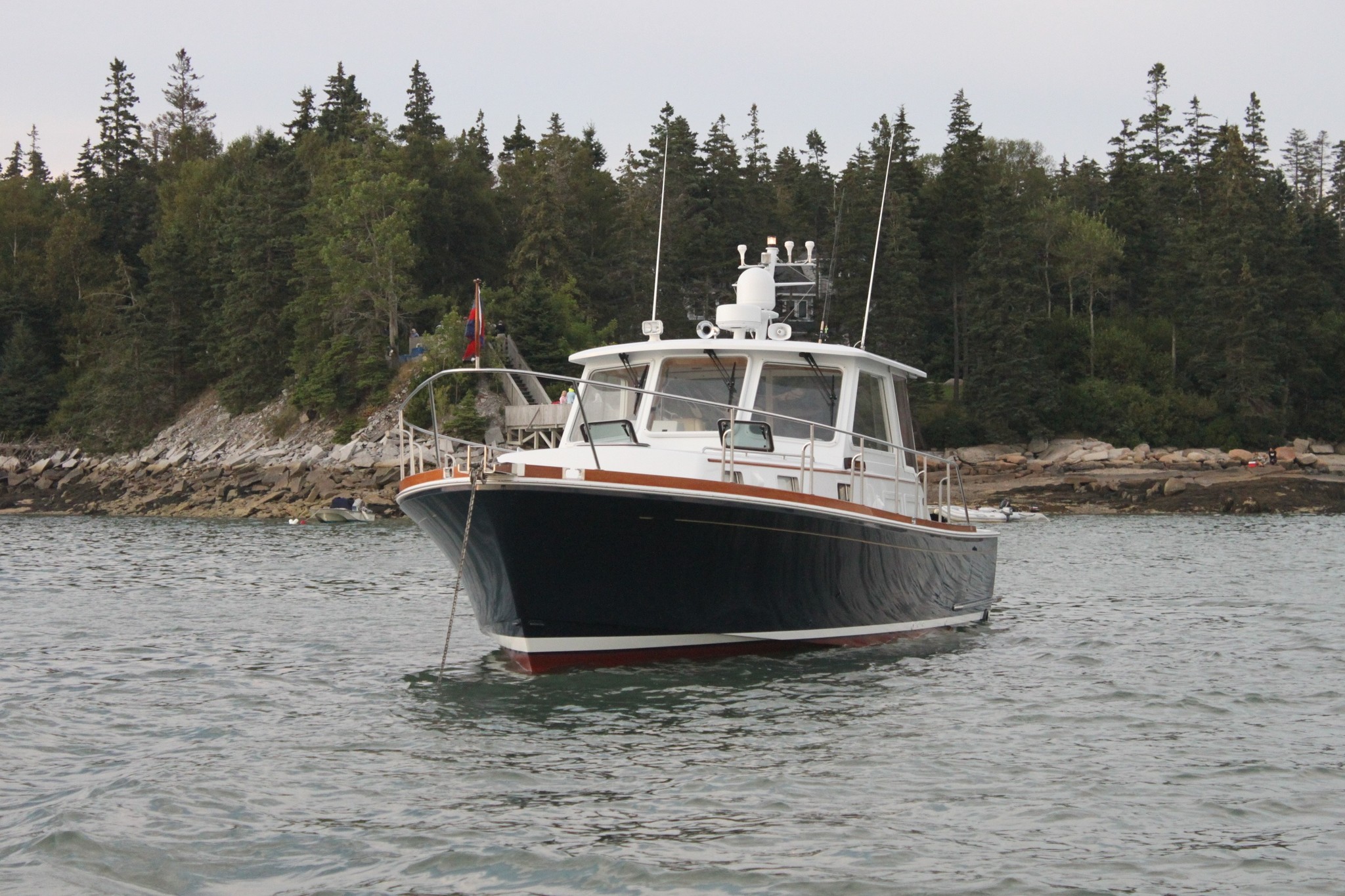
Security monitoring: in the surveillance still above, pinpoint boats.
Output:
[938,495,1051,524]
[387,104,1003,680]
[310,496,376,523]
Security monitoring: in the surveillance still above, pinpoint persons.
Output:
[409,316,506,338]
[559,388,576,404]
[1251,447,1277,467]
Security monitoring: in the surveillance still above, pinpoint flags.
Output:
[462,286,484,361]
